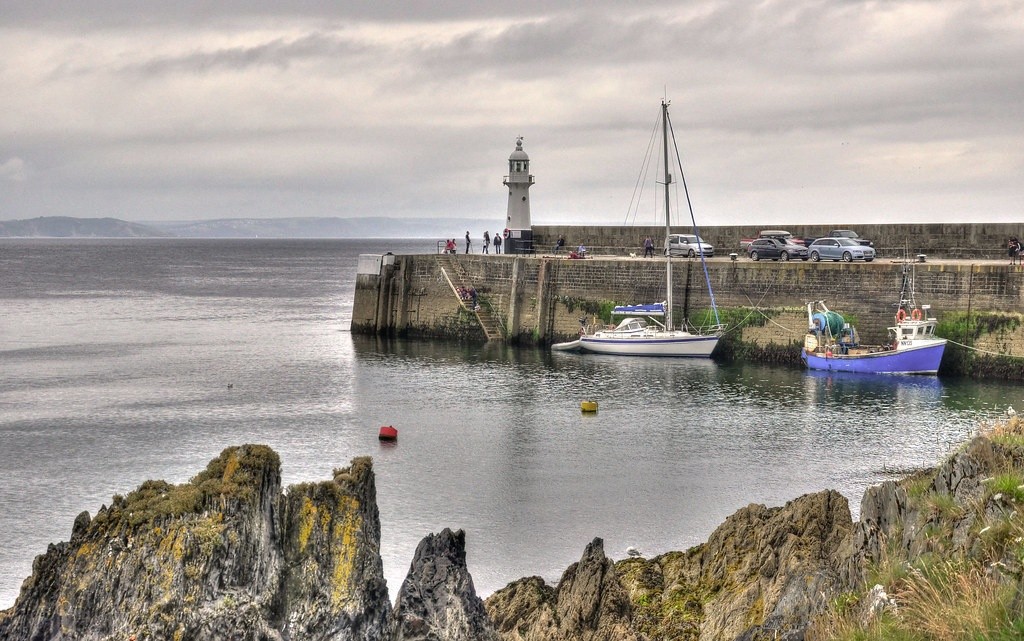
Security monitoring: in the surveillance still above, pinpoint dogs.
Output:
[629,253,637,259]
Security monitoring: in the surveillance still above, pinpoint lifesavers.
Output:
[503,229,509,239]
[911,309,922,322]
[897,309,906,322]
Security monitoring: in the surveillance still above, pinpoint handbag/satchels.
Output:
[651,243,653,248]
[1017,242,1024,251]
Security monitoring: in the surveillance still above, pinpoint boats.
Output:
[800,235,950,375]
[551,338,580,352]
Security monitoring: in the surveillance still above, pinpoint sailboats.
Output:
[579,86,729,359]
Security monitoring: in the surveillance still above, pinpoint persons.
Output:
[465,231,471,254]
[483,231,490,254]
[1010,237,1020,265]
[578,243,586,255]
[494,233,501,254]
[568,251,585,259]
[642,235,655,259]
[445,239,456,254]
[457,287,481,311]
[553,235,565,254]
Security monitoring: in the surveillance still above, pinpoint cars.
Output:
[747,236,809,262]
[662,234,714,258]
[807,237,876,263]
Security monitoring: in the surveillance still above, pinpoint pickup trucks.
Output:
[803,229,874,249]
[739,229,806,253]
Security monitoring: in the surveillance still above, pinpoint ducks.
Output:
[227,383,234,388]
